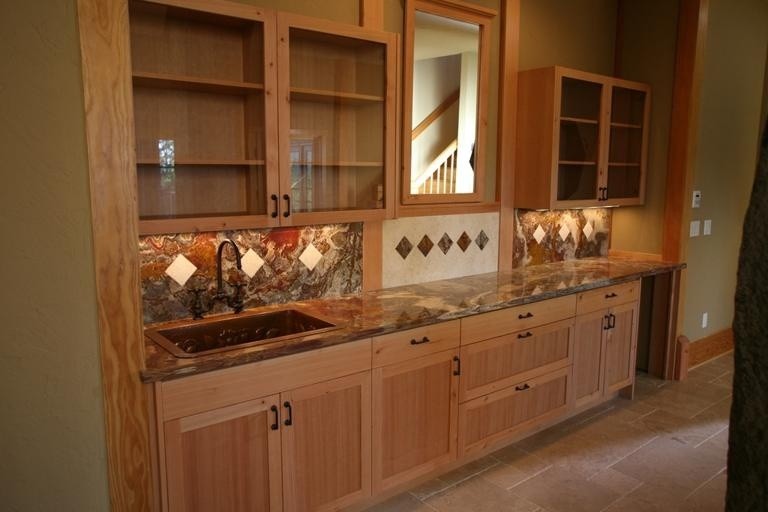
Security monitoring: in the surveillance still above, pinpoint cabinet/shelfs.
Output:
[573,278,642,418]
[370,319,460,503]
[147,337,370,512]
[513,64,652,210]
[78,0,397,236]
[460,294,577,466]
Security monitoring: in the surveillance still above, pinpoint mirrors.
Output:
[402,0,495,205]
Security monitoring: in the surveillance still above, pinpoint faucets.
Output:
[215,238,242,300]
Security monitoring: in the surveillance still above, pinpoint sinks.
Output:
[152,304,339,354]
